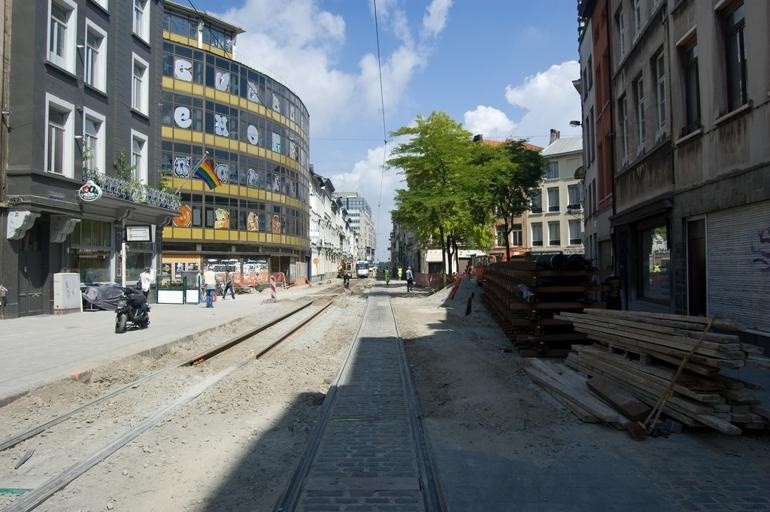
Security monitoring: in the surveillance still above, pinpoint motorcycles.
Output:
[113,281,153,334]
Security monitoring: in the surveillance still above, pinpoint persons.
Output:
[384,265,390,285]
[222,268,236,299]
[343,273,350,289]
[397,265,403,280]
[139,266,152,306]
[406,265,414,292]
[203,265,217,308]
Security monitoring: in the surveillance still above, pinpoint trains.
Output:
[355,260,370,278]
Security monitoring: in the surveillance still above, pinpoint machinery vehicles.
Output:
[467,248,532,279]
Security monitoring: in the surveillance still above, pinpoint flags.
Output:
[195,159,221,190]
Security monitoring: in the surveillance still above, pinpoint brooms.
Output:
[622,348,693,442]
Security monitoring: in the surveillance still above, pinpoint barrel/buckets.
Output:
[554,318,572,341]
